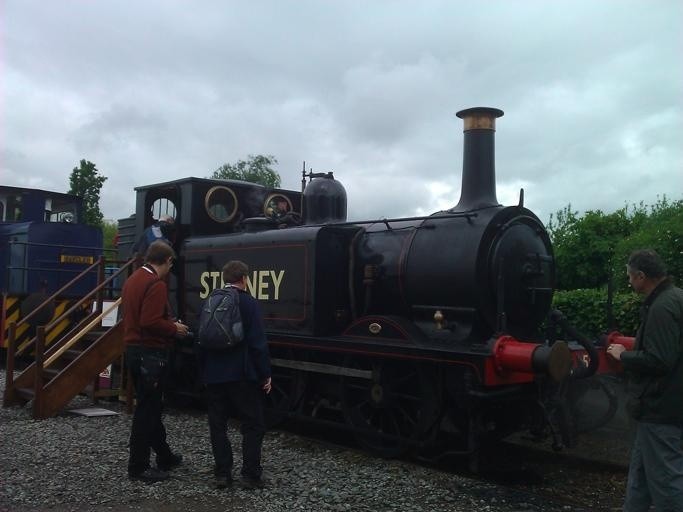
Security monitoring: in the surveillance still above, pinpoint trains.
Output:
[2,108,616,466]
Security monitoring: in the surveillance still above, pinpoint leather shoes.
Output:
[240,472,266,488]
[129,469,168,481]
[218,476,230,489]
[159,452,182,470]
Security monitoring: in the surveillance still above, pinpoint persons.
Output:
[196,260,276,489]
[120,240,189,483]
[606,248,683,512]
[130,214,184,319]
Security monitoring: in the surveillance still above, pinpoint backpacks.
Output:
[198,283,244,347]
